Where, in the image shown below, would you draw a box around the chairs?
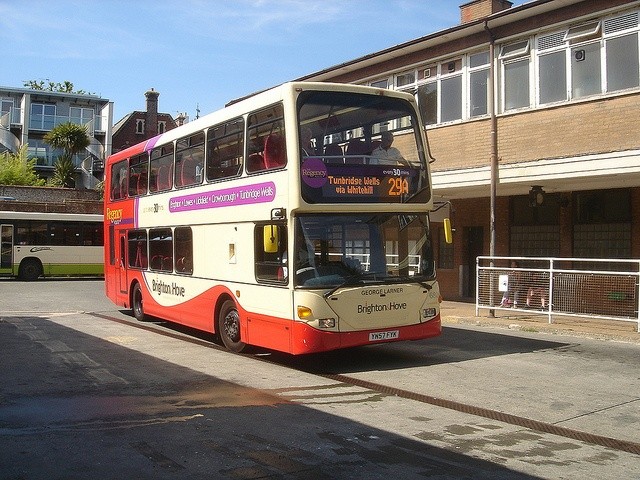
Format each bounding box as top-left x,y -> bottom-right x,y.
278,250 -> 287,280
168,162 -> 174,188
136,171 -> 146,195
247,153 -> 266,171
129,173 -> 139,197
263,133 -> 284,168
128,254 -> 193,272
156,165 -> 170,191
120,177 -> 129,197
111,183 -> 120,199
180,157 -> 194,186
300,127 -> 382,165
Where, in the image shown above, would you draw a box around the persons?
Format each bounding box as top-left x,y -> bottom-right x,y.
370,131 -> 410,165
283,234 -> 311,280
500,260 -> 518,307
299,127 -> 315,154
525,273 -> 546,309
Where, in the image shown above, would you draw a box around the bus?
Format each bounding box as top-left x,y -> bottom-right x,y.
0,210 -> 104,282
103,80 -> 456,358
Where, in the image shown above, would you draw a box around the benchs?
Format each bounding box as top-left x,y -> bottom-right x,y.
498,287 -> 559,311
